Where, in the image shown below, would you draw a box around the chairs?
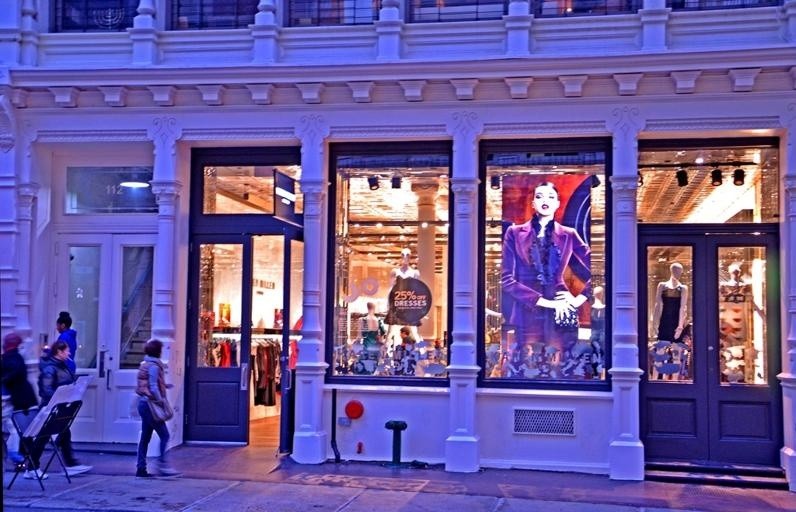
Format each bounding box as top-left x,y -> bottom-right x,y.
7,397 -> 84,492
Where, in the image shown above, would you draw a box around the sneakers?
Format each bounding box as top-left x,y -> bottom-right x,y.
24,468 -> 48,480
63,464 -> 93,476
136,469 -> 183,480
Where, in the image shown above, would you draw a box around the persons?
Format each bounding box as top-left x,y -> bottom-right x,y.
23,310 -> 95,479
0,330 -> 37,463
395,325 -> 418,376
502,181 -> 591,360
373,248 -> 431,379
592,286 -> 606,345
136,338 -> 185,479
719,264 -> 766,342
351,302 -> 388,376
650,263 -> 688,382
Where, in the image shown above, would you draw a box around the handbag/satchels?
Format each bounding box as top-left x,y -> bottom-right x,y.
148,398 -> 173,421
